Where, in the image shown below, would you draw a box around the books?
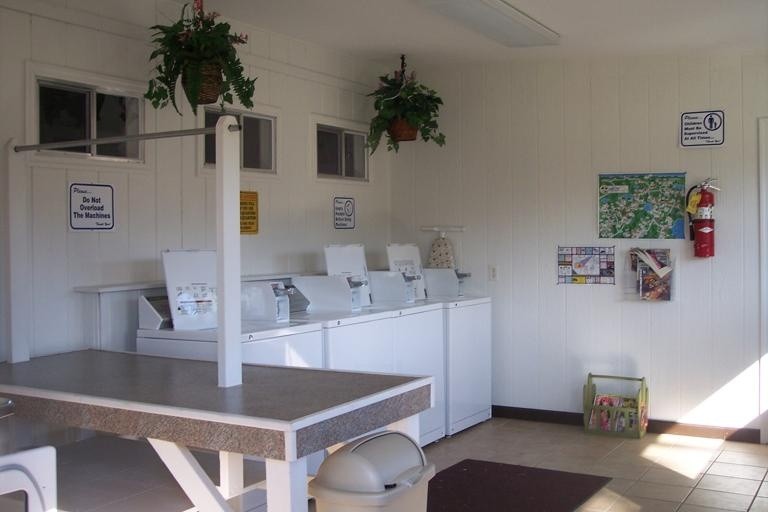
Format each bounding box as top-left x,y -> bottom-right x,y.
615,396 -> 638,431
630,246 -> 673,302
587,394 -> 620,431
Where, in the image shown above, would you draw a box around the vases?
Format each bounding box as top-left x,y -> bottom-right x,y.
386,115 -> 418,142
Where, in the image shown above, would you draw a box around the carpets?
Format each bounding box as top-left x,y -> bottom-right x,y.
427,458 -> 613,512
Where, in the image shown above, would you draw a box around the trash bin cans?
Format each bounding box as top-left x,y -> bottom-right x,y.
307,430 -> 437,512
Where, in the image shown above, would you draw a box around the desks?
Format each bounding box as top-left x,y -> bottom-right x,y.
0,348 -> 436,512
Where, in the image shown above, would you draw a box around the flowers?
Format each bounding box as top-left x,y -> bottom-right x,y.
143,3 -> 258,118
364,64 -> 446,156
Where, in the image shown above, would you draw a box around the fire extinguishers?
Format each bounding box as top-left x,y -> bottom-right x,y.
685,176 -> 721,257
